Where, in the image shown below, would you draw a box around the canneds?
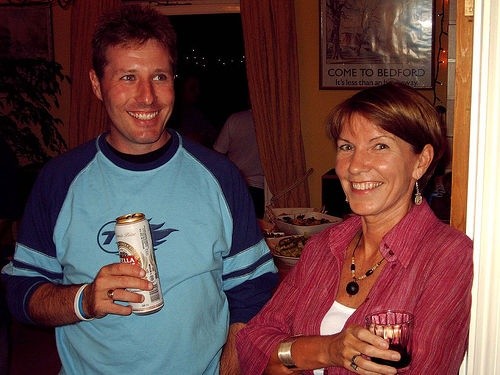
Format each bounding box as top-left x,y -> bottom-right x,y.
115,213 -> 164,314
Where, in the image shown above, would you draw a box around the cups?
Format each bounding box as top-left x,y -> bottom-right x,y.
364,311 -> 415,373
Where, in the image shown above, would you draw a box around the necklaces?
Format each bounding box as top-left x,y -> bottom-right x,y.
347,232 -> 385,297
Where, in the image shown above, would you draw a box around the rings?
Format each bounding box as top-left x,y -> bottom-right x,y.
350,363 -> 359,371
352,354 -> 361,362
107,290 -> 115,300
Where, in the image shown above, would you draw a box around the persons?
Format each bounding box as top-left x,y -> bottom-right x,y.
212,100 -> 265,220
234,82 -> 473,375
1,6 -> 279,375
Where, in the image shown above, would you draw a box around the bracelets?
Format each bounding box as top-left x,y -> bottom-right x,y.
276,335 -> 300,368
73,283 -> 95,321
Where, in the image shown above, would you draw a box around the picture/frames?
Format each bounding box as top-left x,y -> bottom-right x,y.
318,0 -> 435,90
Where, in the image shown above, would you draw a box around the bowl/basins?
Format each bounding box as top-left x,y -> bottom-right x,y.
276,212 -> 344,238
269,235 -> 315,266
268,206 -> 322,217
